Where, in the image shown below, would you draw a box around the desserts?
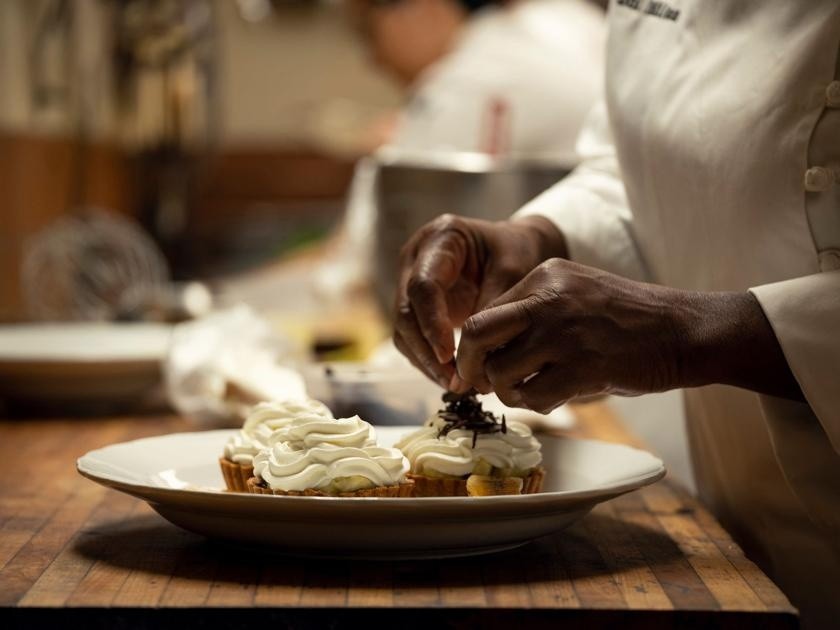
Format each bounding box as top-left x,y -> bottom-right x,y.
219,387 -> 543,498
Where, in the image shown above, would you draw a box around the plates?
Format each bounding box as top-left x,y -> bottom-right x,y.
76,424 -> 668,563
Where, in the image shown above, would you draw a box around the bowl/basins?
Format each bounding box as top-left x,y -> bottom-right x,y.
303,360 -> 437,424
0,320 -> 173,407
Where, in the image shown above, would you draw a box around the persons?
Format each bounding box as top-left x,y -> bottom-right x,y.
389,0 -> 840,630
220,0 -> 692,496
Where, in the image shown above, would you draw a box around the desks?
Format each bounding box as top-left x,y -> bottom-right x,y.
0,334 -> 802,630
